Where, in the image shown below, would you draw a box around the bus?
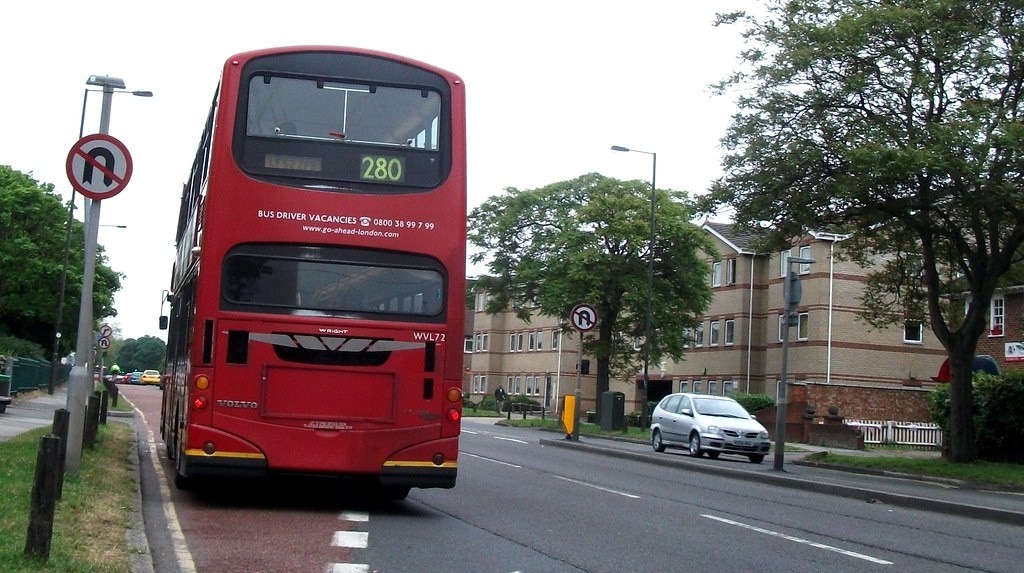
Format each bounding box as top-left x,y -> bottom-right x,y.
159,45 -> 465,507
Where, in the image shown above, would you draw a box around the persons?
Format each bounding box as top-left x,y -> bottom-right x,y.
109,363 -> 120,380
493,384 -> 505,414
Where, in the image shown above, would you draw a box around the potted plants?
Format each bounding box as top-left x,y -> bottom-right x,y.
990,325 -> 1001,335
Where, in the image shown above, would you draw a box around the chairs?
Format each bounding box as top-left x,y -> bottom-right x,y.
279,122 -> 297,135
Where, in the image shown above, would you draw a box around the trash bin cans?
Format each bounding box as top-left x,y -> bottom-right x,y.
602,391 -> 625,433
0,375 -> 11,414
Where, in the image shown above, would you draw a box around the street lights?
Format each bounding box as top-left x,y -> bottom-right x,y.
775,256 -> 817,468
610,145 -> 656,431
64,75 -> 125,474
48,87 -> 152,393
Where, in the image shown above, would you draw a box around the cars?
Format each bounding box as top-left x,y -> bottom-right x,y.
105,374 -> 129,383
129,372 -> 143,383
650,392 -> 772,463
140,369 -> 160,384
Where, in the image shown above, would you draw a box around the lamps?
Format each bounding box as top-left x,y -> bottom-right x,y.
661,362 -> 666,378
